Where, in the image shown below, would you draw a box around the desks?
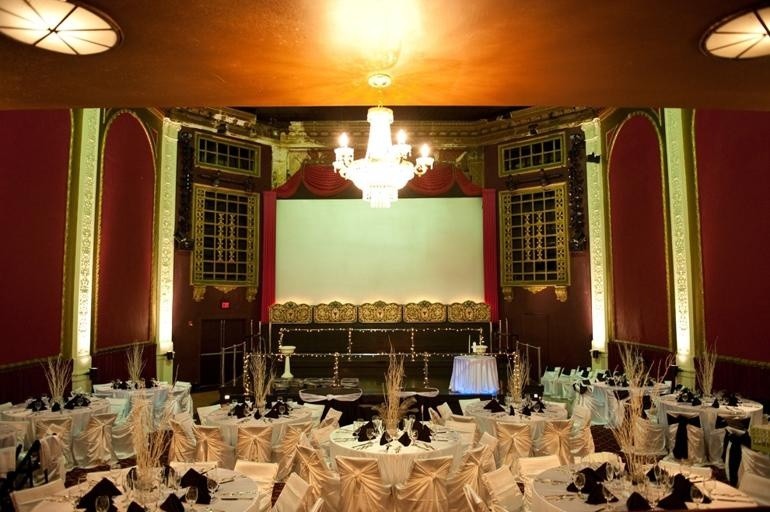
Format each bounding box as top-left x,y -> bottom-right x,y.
447,356 -> 502,395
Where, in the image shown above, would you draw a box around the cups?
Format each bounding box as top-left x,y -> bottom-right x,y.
606,460 -> 614,482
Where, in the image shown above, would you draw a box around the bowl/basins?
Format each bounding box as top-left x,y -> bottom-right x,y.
279,346 -> 296,353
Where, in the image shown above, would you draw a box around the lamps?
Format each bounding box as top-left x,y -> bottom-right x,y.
0,0 -> 125,58
697,1 -> 770,62
332,73 -> 436,212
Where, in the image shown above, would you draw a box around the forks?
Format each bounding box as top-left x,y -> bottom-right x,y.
535,464 -> 576,504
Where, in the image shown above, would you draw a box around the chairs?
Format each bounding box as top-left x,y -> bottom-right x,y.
0,365 -> 770,511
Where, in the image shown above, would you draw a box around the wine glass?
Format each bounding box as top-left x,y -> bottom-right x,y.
602,482 -> 614,512
573,472 -> 586,499
22,377 -> 159,416
467,391 -> 561,417
333,415 -> 453,451
228,396 -> 294,422
45,457 -> 256,511
618,457 -> 750,512
